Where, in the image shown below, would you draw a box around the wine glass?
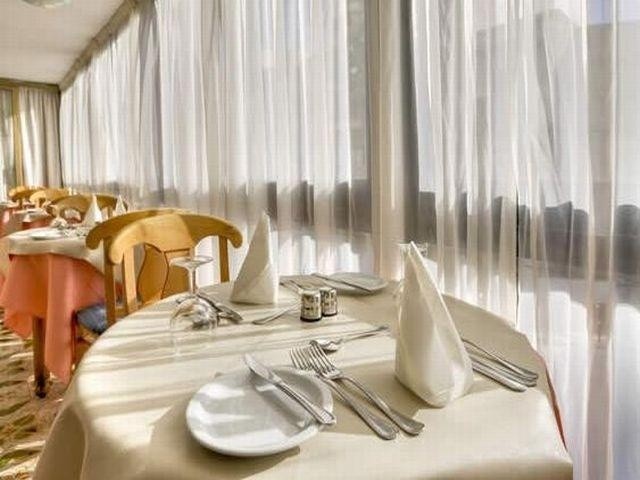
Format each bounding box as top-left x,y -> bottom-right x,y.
48,204 -> 69,230
167,254 -> 217,353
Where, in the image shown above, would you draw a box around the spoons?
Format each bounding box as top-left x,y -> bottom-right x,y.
307,325 -> 390,349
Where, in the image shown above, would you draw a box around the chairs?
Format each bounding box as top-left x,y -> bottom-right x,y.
110,214 -> 244,320
0,182 -> 127,397
69,208 -> 195,366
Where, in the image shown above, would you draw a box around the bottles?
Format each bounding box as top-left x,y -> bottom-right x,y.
298,287 -> 339,323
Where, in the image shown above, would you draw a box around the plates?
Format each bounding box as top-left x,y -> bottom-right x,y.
184,365 -> 335,458
31,230 -> 60,239
324,272 -> 386,296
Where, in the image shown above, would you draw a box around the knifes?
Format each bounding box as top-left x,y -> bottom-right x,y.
244,353 -> 337,428
196,292 -> 245,322
315,272 -> 373,293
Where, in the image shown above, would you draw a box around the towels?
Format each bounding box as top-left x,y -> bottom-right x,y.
394,241 -> 474,408
231,210 -> 283,307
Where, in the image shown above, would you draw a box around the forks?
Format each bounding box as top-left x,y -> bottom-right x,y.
302,342 -> 426,436
287,349 -> 399,440
